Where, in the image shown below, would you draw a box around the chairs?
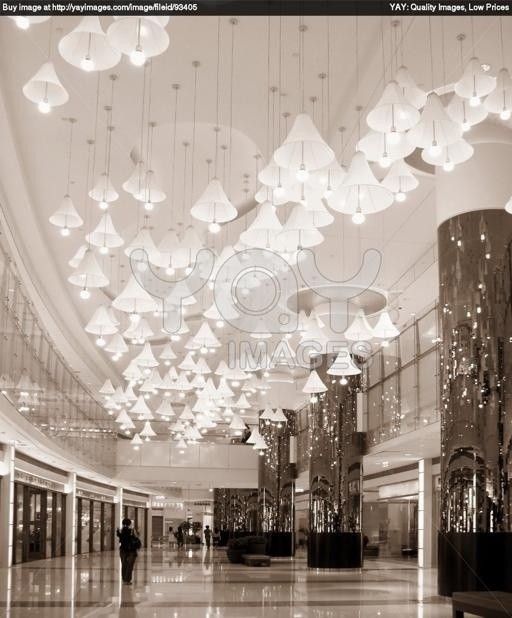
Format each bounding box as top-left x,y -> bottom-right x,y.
363,545 -> 378,557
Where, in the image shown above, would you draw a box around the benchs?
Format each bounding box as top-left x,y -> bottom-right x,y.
452,590 -> 512,618
243,554 -> 272,567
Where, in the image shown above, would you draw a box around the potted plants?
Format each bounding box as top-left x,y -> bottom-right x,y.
226,535 -> 267,562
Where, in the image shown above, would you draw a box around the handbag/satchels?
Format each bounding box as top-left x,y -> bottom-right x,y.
129,537 -> 140,550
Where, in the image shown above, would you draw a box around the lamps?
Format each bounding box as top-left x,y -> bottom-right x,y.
21,12 -> 512,458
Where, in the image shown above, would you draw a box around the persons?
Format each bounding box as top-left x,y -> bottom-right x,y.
203,525 -> 212,549
167,525 -> 176,551
119,518 -> 142,585
212,526 -> 222,549
176,525 -> 184,548
